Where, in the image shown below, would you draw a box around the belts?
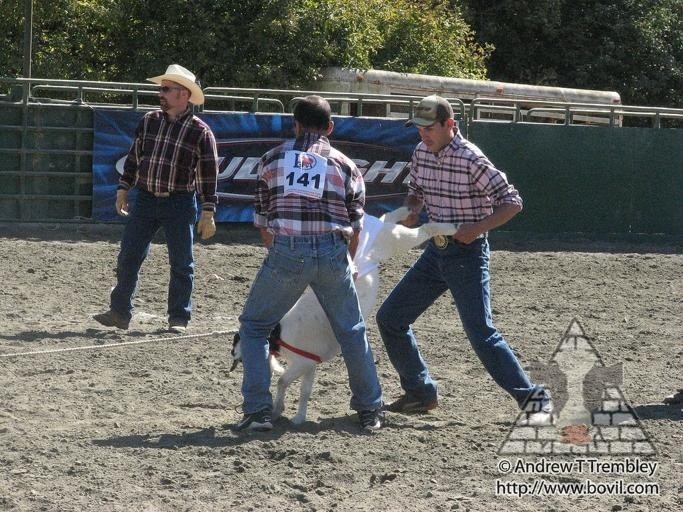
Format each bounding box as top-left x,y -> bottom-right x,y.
139,188 -> 184,199
431,236 -> 456,250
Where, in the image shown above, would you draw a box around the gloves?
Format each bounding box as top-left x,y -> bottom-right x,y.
197,211 -> 216,240
116,191 -> 128,216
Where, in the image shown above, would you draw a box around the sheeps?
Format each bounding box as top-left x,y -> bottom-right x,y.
230,205 -> 486,426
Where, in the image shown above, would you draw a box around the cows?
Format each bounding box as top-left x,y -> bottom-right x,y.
529,357 -> 624,446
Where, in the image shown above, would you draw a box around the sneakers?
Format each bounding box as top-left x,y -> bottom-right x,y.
93,311 -> 129,330
233,409 -> 273,432
360,402 -> 385,431
388,397 -> 438,412
170,323 -> 186,334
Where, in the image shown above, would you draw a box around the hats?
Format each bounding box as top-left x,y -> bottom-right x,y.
146,64 -> 205,106
404,94 -> 453,127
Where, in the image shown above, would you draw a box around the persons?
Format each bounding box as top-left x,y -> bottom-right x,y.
233,94 -> 384,432
375,93 -> 554,426
93,63 -> 217,333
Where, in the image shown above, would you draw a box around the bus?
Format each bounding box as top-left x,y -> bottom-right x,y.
296,63 -> 627,129
